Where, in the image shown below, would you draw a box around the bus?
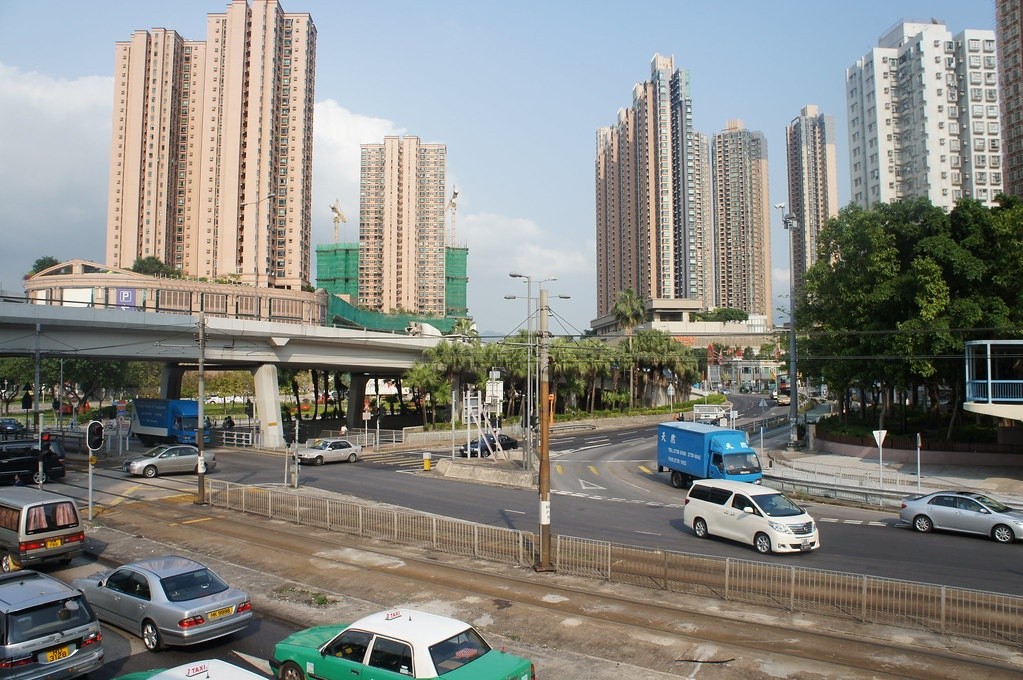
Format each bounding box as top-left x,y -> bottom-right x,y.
776,372 -> 790,405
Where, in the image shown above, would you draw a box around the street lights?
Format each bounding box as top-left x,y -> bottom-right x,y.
504,272 -> 570,468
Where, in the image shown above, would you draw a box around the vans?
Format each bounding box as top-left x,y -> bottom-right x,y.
0,485 -> 84,573
0,439 -> 66,484
683,479 -> 821,554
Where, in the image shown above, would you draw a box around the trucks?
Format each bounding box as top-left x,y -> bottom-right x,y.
655,422 -> 764,490
132,397 -> 210,448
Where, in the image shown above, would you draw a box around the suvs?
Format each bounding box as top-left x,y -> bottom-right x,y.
0,568 -> 103,678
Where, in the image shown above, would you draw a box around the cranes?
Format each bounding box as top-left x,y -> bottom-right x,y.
444,184 -> 460,245
327,199 -> 346,243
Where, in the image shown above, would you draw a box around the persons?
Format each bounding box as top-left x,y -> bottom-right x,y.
222,416 -> 235,431
71,420 -> 74,430
720,457 -> 735,471
169,449 -> 176,457
960,499 -> 979,512
204,416 -> 210,428
341,423 -> 348,436
109,417 -> 117,430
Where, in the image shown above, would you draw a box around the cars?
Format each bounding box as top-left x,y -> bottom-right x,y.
72,554 -> 536,680
898,490 -> 1023,544
121,444 -> 216,478
293,438 -> 361,466
0,418 -> 23,434
459,434 -> 519,458
697,407 -> 726,426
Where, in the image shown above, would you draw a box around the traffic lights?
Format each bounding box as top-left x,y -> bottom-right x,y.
41,431 -> 51,458
86,421 -> 104,451
530,416 -> 539,429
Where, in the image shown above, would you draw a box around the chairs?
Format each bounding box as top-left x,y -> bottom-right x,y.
166,582 -> 177,592
16,617 -> 34,634
57,608 -> 73,621
197,575 -> 209,586
